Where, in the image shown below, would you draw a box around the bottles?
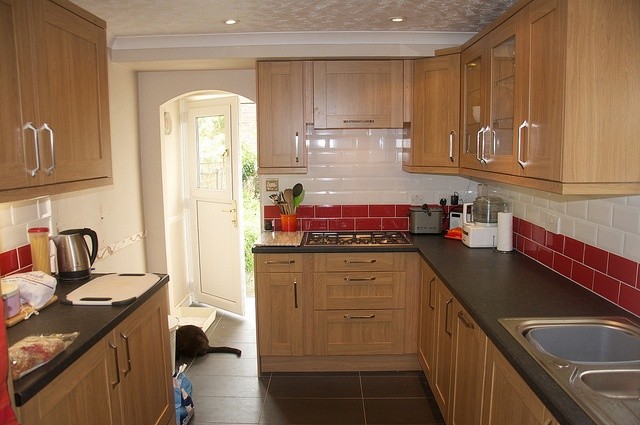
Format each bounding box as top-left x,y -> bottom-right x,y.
265,220 -> 272,230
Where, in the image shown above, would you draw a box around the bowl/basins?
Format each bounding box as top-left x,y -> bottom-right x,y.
472,106 -> 481,123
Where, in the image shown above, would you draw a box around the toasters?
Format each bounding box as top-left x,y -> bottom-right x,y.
409,204 -> 443,236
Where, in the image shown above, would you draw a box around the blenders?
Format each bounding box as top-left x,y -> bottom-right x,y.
462,184 -> 504,249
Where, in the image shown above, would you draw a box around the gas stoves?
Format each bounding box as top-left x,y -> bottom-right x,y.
301,232 -> 413,248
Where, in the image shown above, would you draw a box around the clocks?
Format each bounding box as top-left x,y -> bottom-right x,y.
164,112 -> 173,134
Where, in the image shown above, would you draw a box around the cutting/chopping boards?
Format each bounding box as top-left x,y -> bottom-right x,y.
5,294 -> 58,327
58,273 -> 162,305
254,230 -> 304,247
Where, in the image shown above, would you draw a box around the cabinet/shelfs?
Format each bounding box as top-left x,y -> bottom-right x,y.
517,1 -> 640,195
303,253 -> 418,372
438,276 -> 454,425
255,254 -> 303,378
418,252 -> 437,405
1,1 -> 113,204
487,336 -> 560,424
454,298 -> 487,425
313,61 -> 403,129
17,284 -> 176,424
403,46 -> 461,174
461,1 -> 517,185
256,58 -> 313,175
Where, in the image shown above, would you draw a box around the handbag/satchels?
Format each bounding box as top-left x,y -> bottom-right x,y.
172,364 -> 194,425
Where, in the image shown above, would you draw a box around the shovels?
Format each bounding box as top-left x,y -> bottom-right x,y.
284,189 -> 294,214
294,190 -> 306,214
293,183 -> 303,197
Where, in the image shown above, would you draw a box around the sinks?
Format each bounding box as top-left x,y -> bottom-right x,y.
577,367 -> 640,400
517,320 -> 640,363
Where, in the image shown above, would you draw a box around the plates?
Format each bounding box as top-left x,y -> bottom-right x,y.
444,235 -> 462,240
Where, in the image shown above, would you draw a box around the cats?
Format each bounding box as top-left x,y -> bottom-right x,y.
175,324 -> 242,361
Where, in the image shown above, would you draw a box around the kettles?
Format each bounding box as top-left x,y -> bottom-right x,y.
49,228 -> 98,281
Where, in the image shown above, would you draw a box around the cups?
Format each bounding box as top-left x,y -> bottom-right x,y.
28,228 -> 51,275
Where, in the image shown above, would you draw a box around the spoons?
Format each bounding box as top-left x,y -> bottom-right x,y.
276,201 -> 289,214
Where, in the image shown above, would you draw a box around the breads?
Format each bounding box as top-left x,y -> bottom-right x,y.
20,335 -> 64,362
9,345 -> 34,378
